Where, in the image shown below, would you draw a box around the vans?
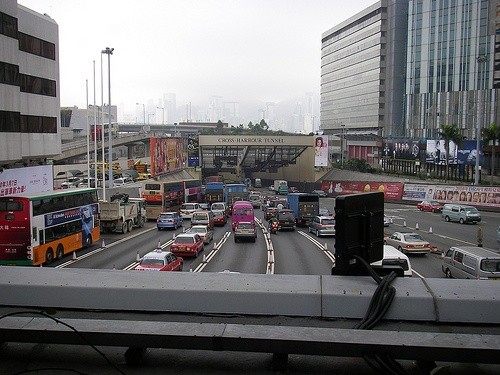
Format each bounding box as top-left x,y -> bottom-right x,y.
371,244 -> 412,279
113,177 -> 135,187
179,202 -> 203,219
190,211 -> 216,230
441,245 -> 500,281
442,203 -> 481,224
211,202 -> 229,218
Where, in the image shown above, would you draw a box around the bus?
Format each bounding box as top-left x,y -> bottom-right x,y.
286,192 -> 320,227
230,200 -> 255,230
134,162 -> 148,180
141,178 -> 201,219
0,187 -> 102,267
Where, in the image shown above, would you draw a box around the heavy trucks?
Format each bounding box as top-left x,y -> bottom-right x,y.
89,162 -> 121,181
206,181 -> 224,202
226,184 -> 250,213
99,196 -> 147,235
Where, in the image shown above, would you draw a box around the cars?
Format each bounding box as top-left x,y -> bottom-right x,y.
385,231 -> 431,256
61,176 -> 98,189
234,221 -> 256,243
245,177 -> 393,237
214,212 -> 228,226
135,248 -> 184,272
200,204 -> 212,211
417,199 -> 445,214
170,225 -> 213,258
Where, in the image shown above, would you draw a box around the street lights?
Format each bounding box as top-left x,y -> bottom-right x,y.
148,113 -> 155,124
101,46 -> 115,189
475,52 -> 490,186
136,102 -> 146,124
157,106 -> 165,124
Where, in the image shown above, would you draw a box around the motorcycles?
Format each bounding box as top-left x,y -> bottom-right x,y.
268,221 -> 278,234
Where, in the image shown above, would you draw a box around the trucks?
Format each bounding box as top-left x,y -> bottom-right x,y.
273,179 -> 289,195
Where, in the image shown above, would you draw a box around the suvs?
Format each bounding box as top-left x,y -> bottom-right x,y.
156,211 -> 184,230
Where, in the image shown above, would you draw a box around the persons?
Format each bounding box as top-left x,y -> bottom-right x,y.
428,189 -> 500,203
268,215 -> 278,230
432,141 -> 440,163
394,142 -> 409,156
315,137 -> 323,156
